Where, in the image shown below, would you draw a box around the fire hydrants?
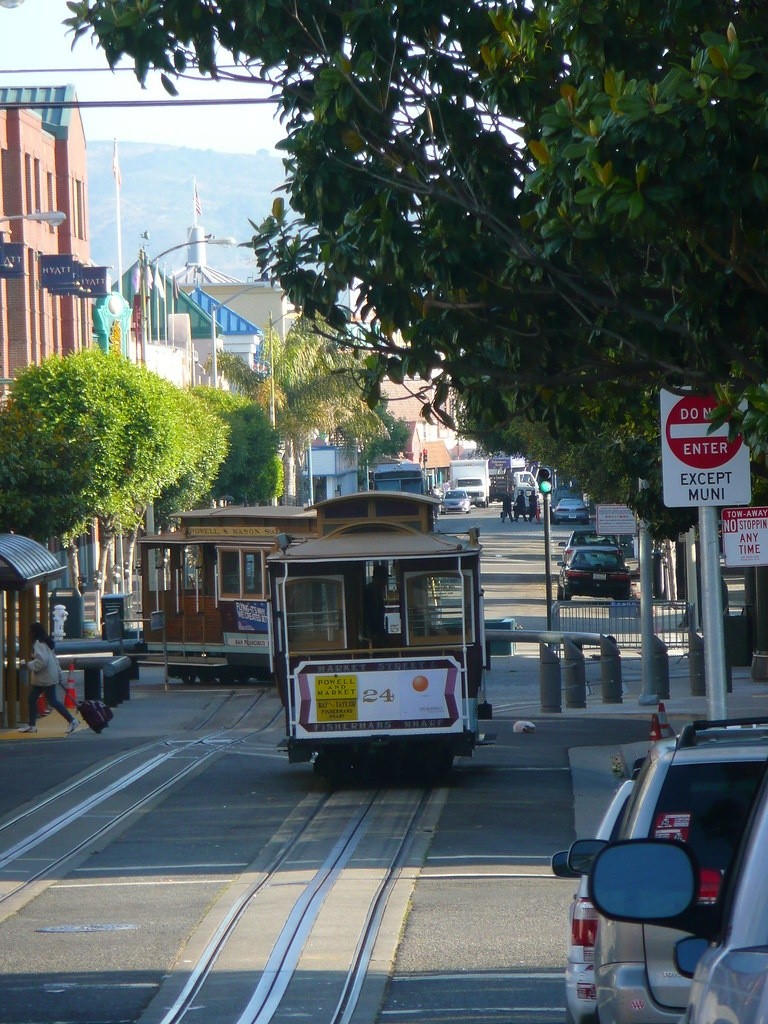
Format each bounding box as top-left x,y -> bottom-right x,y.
51,604 -> 69,641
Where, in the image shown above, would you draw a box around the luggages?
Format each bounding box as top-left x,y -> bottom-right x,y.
59,683 -> 113,734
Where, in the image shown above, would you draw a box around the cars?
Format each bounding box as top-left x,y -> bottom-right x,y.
513,487 -> 590,524
551,768 -> 641,1024
557,546 -> 631,601
557,529 -> 625,563
587,773 -> 766,1024
441,490 -> 472,515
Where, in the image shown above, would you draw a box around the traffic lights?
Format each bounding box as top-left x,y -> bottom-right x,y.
423,450 -> 427,463
538,467 -> 553,494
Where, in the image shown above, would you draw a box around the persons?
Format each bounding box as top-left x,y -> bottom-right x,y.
18,621 -> 80,734
502,490 -> 539,523
364,566 -> 391,658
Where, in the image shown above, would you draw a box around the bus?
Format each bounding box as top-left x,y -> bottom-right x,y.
136,491 -> 492,786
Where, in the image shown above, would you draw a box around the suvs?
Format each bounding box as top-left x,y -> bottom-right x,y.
567,714 -> 768,1024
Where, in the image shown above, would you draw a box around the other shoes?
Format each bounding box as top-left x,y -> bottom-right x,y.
64,718 -> 81,733
17,726 -> 37,733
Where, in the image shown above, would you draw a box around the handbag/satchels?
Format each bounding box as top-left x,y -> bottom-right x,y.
513,502 -> 517,511
500,512 -> 507,518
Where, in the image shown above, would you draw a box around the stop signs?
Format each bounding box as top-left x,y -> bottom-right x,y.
665,396 -> 743,470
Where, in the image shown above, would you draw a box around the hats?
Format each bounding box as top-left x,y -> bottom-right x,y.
371,565 -> 392,578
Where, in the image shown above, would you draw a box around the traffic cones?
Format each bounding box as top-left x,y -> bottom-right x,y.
63,663 -> 78,708
658,703 -> 675,738
38,692 -> 47,715
649,713 -> 664,751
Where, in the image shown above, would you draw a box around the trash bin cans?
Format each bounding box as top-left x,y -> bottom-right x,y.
48,588 -> 85,638
101,592 -> 131,639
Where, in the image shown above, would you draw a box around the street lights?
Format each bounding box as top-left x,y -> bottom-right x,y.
211,283 -> 281,387
142,238 -> 236,364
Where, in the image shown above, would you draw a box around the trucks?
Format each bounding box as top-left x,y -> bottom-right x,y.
369,464 -> 426,495
449,459 -> 539,508
